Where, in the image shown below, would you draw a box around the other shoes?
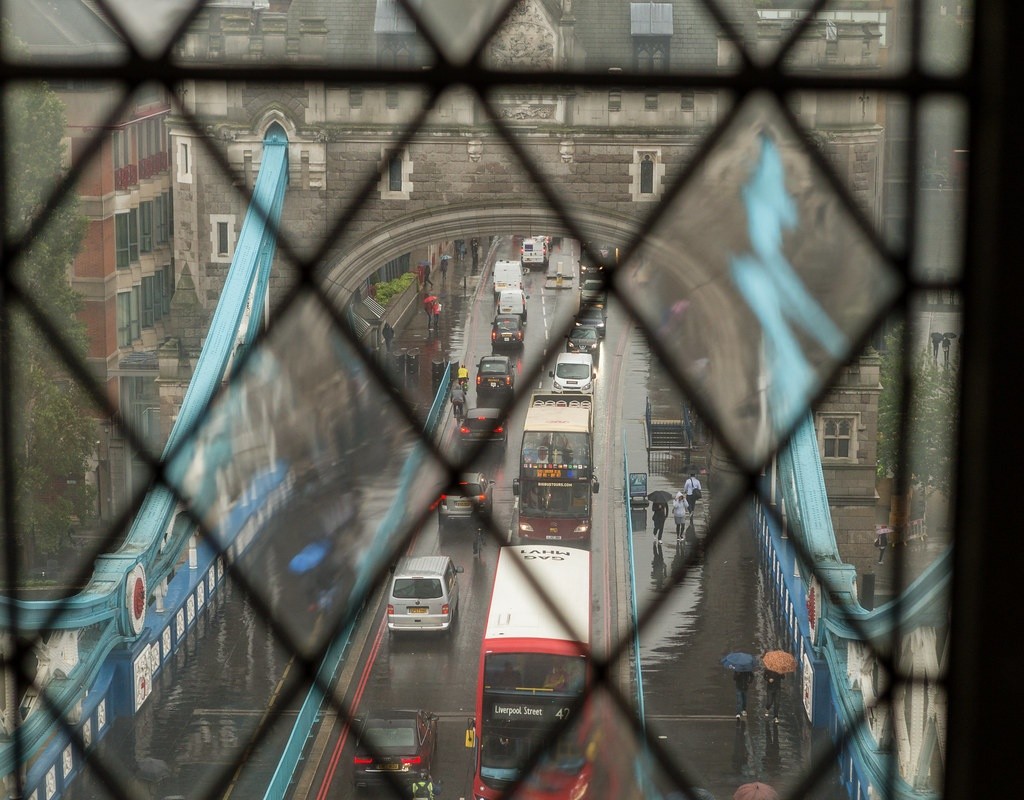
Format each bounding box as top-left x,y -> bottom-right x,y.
460,417 -> 463,422
453,413 -> 456,418
654,535 -> 656,540
431,284 -> 433,287
690,512 -> 693,518
736,714 -> 741,717
680,535 -> 683,539
659,539 -> 663,544
765,709 -> 768,716
424,283 -> 426,286
774,717 -> 779,723
742,711 -> 747,716
677,536 -> 679,540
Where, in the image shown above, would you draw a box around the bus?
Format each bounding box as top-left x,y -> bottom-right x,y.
513,392 -> 599,545
465,545 -> 601,800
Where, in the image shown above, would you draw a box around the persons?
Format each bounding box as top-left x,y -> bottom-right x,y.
457,364 -> 469,391
684,473 -> 701,515
441,259 -> 448,279
416,264 -> 434,288
878,533 -> 887,565
382,322 -> 394,353
525,485 -> 539,509
534,446 -> 553,463
763,669 -> 786,724
493,661 -> 522,689
541,665 -> 566,691
489,721 -> 515,767
732,671 -> 755,719
405,768 -> 442,800
455,239 -> 466,262
470,237 -> 478,256
450,383 -> 466,418
672,490 -> 690,541
425,300 -> 442,328
651,501 -> 669,544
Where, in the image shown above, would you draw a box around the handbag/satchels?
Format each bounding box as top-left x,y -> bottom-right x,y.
693,489 -> 702,500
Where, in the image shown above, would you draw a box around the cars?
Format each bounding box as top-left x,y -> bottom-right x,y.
491,315 -> 527,348
351,707 -> 440,789
563,251 -> 609,356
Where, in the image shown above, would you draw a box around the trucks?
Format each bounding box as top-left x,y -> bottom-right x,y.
491,260 -> 527,295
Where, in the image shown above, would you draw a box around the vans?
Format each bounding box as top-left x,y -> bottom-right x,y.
519,237 -> 549,268
496,289 -> 531,318
547,351 -> 597,397
386,556 -> 464,638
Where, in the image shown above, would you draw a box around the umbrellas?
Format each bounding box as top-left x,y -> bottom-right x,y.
678,463 -> 711,476
440,254 -> 452,259
876,528 -> 895,534
733,781 -> 779,800
666,786 -> 715,800
415,258 -> 432,266
648,490 -> 673,503
762,650 -> 797,673
423,295 -> 438,304
721,652 -> 761,672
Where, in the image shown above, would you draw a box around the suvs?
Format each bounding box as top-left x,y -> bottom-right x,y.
457,407 -> 510,453
438,472 -> 496,527
475,355 -> 516,398
